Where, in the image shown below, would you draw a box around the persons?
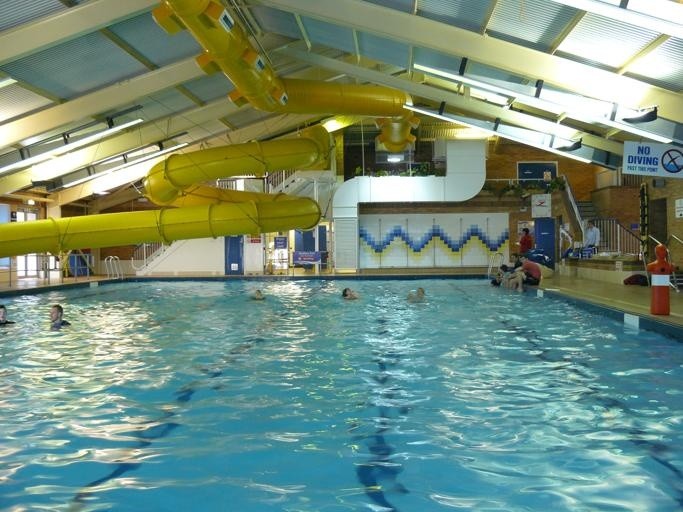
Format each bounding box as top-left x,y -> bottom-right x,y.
582,220 -> 601,254
647,244 -> 675,316
0,304 -> 16,328
255,289 -> 264,300
490,253 -> 541,293
407,286 -> 426,302
519,229 -> 532,253
342,287 -> 358,300
49,305 -> 71,330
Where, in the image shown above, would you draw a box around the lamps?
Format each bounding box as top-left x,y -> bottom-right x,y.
554,139 -> 581,151
622,107 -> 657,124
